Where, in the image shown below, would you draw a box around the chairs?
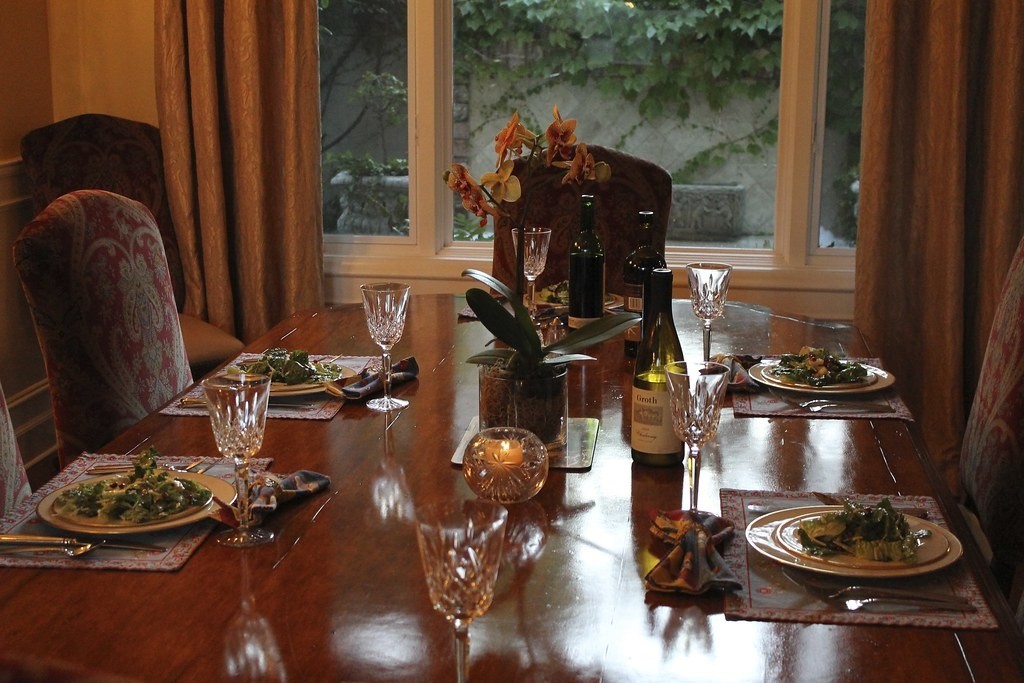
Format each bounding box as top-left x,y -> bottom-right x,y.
12,189 -> 195,465
489,146 -> 670,302
956,233 -> 1024,615
23,114 -> 243,378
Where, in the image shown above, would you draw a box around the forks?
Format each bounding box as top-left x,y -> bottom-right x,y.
781,565 -> 977,611
88,456 -> 221,474
767,388 -> 896,413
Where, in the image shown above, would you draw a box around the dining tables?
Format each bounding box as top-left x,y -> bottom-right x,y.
0,289 -> 1023,683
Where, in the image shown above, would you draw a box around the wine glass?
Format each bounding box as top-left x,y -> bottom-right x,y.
201,373 -> 274,544
686,262 -> 732,364
359,282 -> 411,411
664,361 -> 730,514
413,499 -> 507,683
511,227 -> 552,326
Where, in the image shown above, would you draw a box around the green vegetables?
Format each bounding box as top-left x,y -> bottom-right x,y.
793,494 -> 932,561
50,443 -> 212,523
226,346 -> 341,385
768,347 -> 868,387
537,277 -> 615,306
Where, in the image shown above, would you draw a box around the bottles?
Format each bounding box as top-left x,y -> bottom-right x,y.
631,269 -> 687,465
530,195 -> 667,357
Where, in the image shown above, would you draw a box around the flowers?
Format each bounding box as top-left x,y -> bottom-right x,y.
443,106 -> 642,377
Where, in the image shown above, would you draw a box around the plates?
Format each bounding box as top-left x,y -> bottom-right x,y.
522,291 -> 626,309
269,360 -> 356,397
745,506 -> 963,576
37,469 -> 238,534
749,360 -> 896,393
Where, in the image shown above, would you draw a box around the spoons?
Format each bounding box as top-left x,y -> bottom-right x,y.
0,539 -> 106,556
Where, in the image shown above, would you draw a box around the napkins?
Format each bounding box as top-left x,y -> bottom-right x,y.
642,509 -> 742,594
322,355 -> 419,402
209,468 -> 330,532
710,351 -> 765,392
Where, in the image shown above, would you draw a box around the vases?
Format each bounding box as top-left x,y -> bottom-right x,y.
479,361 -> 570,462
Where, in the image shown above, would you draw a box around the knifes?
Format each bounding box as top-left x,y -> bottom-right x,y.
0,533 -> 168,553
180,397 -> 313,409
